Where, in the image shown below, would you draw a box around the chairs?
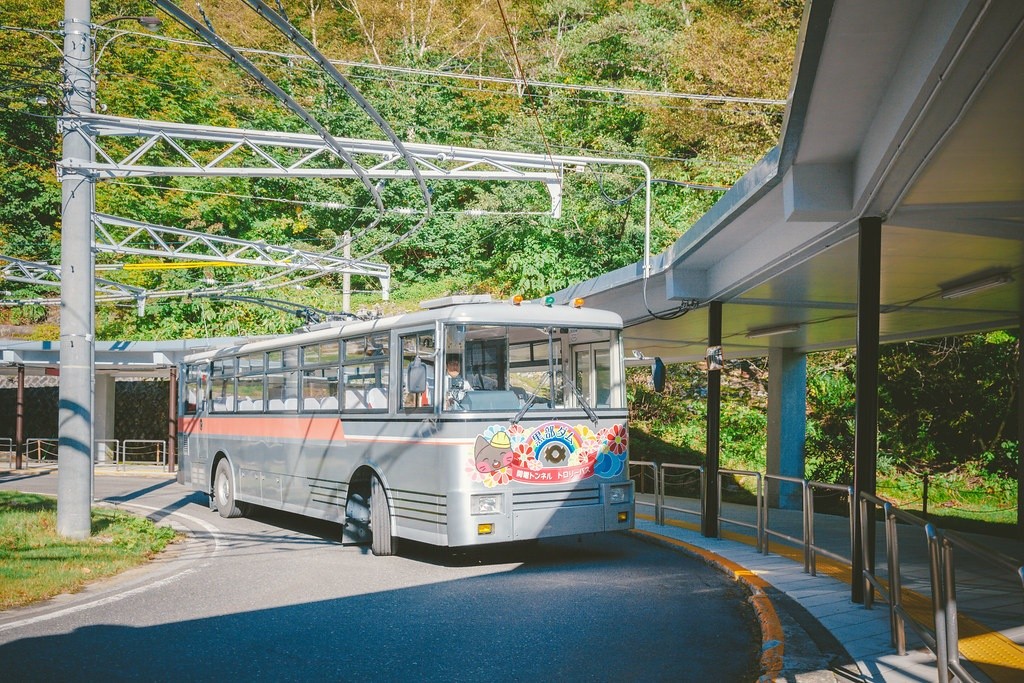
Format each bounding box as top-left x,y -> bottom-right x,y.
212,387 -> 388,413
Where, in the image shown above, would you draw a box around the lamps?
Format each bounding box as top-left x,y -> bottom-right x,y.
942,274 -> 1016,300
746,326 -> 800,339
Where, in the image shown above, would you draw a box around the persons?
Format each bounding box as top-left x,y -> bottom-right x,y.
444,358 -> 474,411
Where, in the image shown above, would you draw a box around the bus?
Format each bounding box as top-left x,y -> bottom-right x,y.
176,294 -> 667,557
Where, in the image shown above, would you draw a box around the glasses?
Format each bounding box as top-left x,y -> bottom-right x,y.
447,362 -> 460,366
